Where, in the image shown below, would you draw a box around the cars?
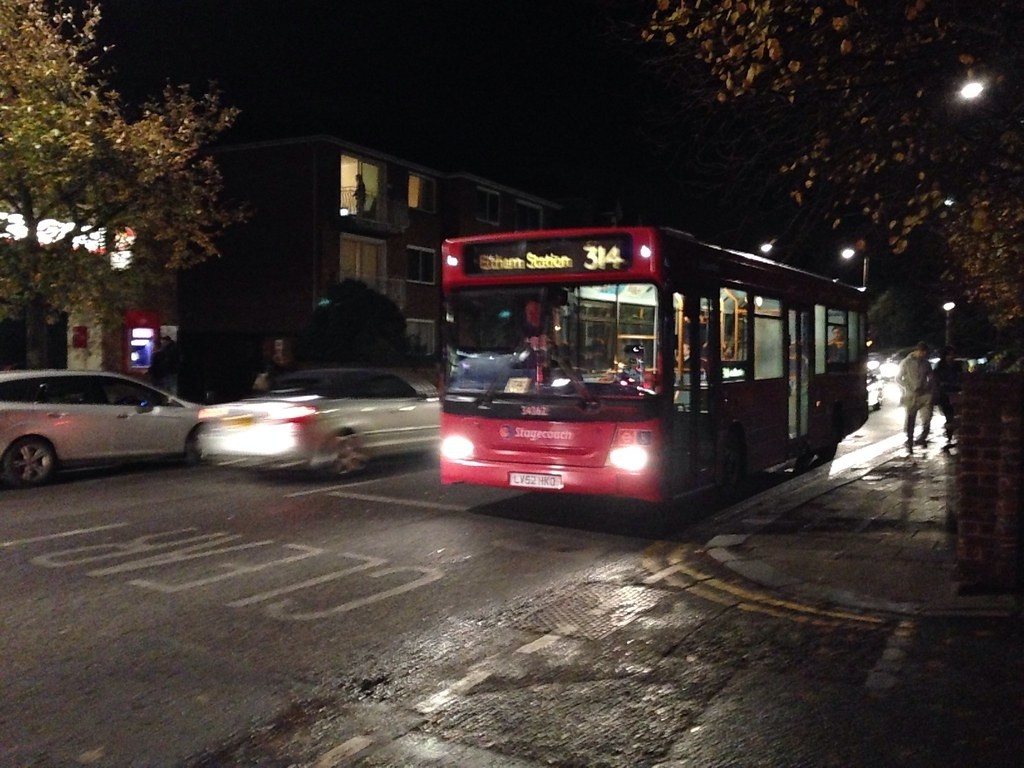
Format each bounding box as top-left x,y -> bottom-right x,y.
199,369 -> 439,477
0,370 -> 207,489
866,344 -> 975,411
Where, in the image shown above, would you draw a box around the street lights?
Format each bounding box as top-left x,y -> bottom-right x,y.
942,301 -> 955,346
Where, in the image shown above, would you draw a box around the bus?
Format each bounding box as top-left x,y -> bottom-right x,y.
439,226 -> 869,504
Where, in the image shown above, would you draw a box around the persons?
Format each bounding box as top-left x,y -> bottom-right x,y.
145,336 -> 189,401
932,345 -> 971,441
353,174 -> 367,213
828,326 -> 846,348
902,342 -> 935,453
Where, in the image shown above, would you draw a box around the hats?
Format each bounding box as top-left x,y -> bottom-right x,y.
916,342 -> 929,350
